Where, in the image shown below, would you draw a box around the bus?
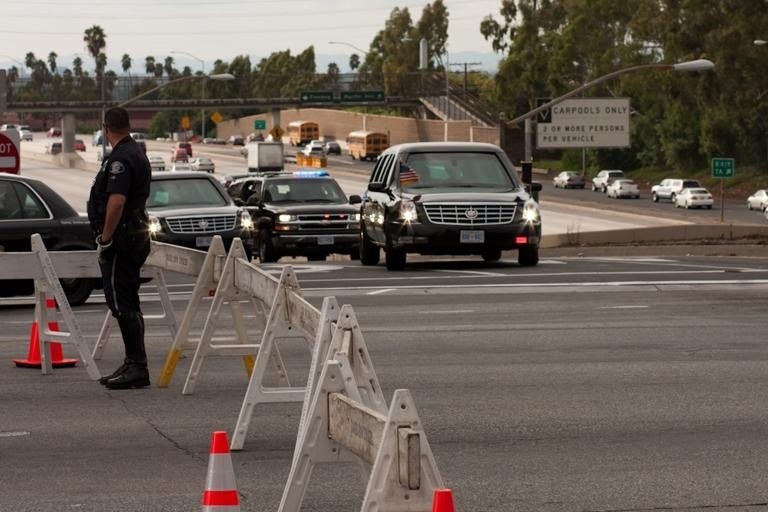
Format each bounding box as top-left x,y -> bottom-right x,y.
287,120 -> 318,146
344,128 -> 388,161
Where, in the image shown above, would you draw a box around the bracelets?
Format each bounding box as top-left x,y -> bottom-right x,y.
95,234 -> 113,247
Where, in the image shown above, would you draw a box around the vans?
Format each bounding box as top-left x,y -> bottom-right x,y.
130,132 -> 144,144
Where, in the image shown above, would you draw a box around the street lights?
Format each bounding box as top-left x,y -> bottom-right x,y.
171,50 -> 204,143
498,58 -> 714,202
399,37 -> 450,119
328,41 -> 368,56
102,73 -> 235,163
754,39 -> 768,46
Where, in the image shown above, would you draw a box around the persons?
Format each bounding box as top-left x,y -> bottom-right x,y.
86,107 -> 151,389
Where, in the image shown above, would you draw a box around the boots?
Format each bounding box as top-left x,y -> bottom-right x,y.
101,312 -> 150,387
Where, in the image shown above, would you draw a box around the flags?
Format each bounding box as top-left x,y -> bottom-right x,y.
399,159 -> 419,186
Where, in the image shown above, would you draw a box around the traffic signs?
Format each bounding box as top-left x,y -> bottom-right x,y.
300,92 -> 333,103
712,157 -> 732,177
254,119 -> 265,129
341,91 -> 384,104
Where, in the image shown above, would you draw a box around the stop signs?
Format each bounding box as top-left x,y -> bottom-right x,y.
0,130 -> 20,174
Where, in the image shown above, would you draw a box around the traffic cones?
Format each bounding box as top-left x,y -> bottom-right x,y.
431,489 -> 454,512
199,431 -> 239,512
12,294 -> 80,365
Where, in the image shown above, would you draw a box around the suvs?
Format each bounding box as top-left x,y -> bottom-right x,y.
219,170 -> 361,261
144,171 -> 254,264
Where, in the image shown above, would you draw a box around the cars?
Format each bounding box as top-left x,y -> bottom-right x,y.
359,141 -> 540,270
227,132 -> 284,171
300,139 -> 341,156
2,124 -> 217,173
552,169 -> 767,219
0,175 -> 153,305
